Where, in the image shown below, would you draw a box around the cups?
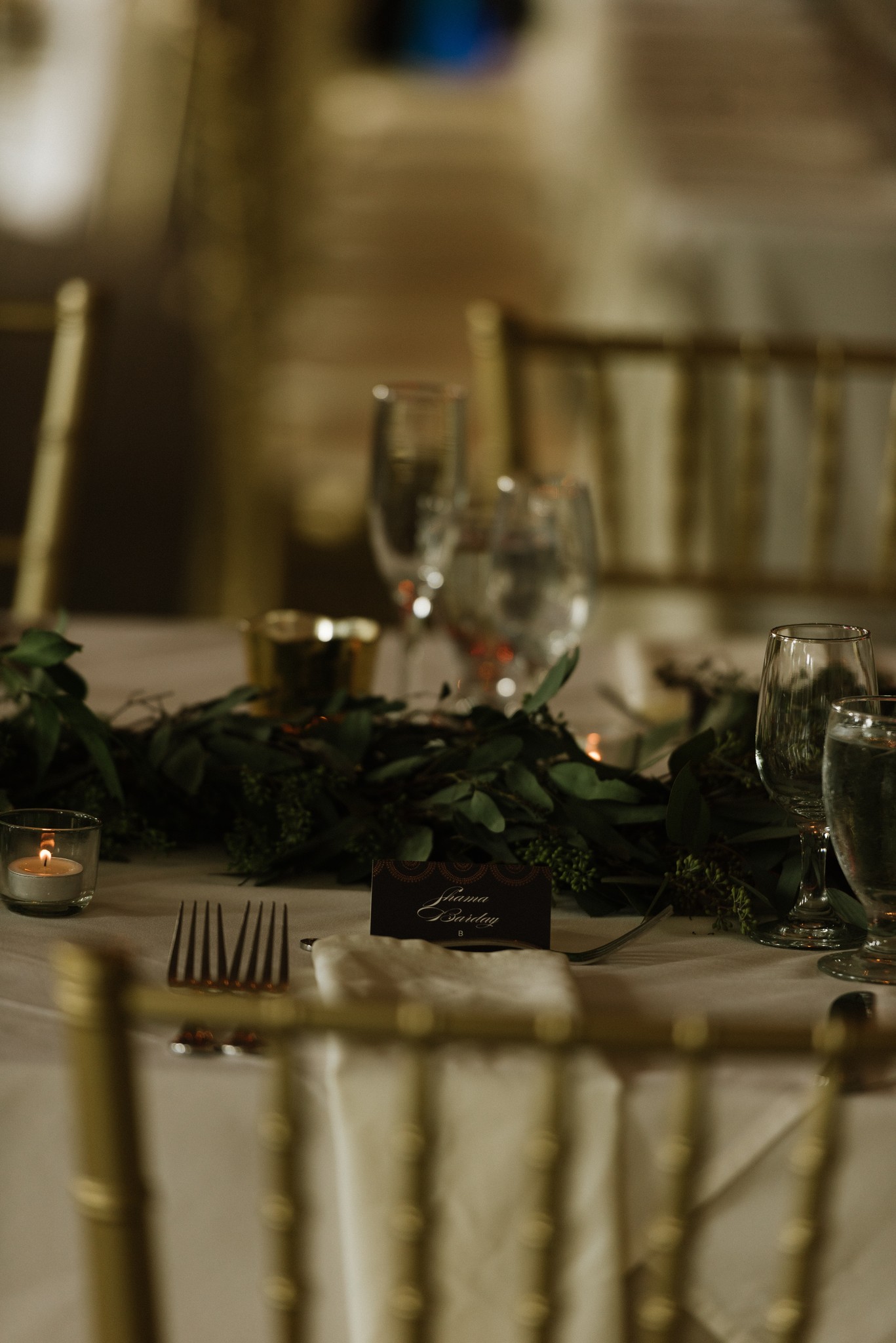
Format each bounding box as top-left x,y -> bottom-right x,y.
0,808 -> 103,918
247,604 -> 382,711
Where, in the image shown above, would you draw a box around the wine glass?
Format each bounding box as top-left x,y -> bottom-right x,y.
817,697 -> 896,984
366,384 -> 467,716
446,478 -> 606,719
749,621 -> 878,951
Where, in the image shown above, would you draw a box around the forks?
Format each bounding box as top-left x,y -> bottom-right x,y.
169,898 -> 230,1058
226,901 -> 293,1058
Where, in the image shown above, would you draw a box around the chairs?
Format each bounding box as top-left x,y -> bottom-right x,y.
52,928 -> 877,1343
461,294 -> 893,666
